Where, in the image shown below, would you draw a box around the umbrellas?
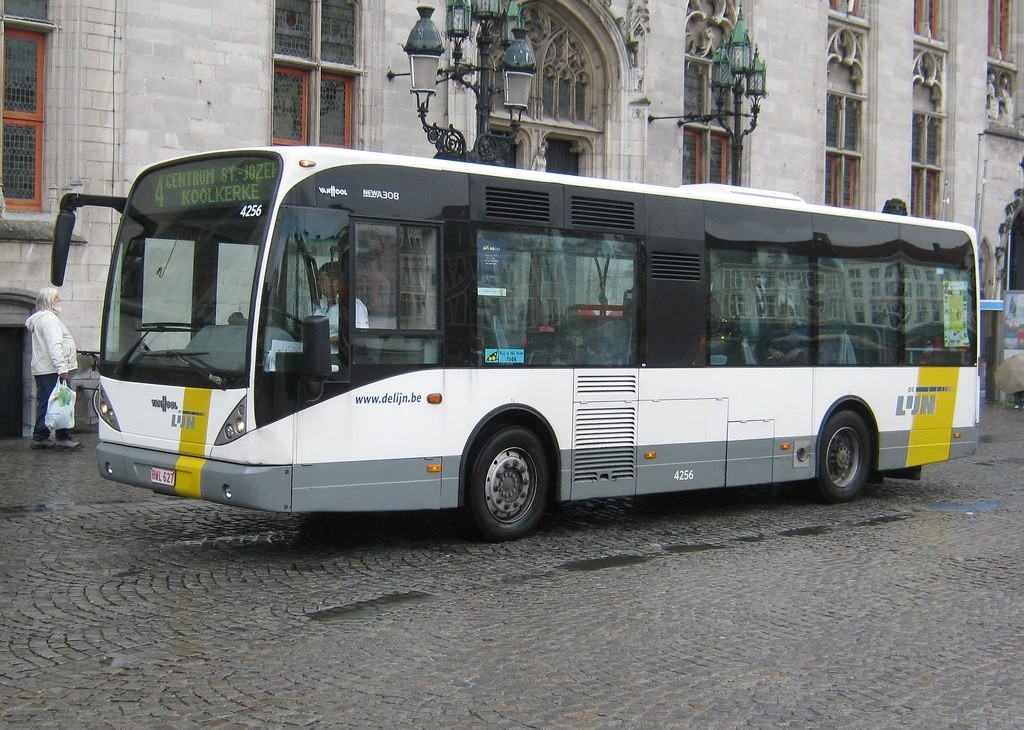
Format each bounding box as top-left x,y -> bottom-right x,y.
994,353 -> 1024,394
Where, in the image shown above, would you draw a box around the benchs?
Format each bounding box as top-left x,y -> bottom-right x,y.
563,315 -> 633,366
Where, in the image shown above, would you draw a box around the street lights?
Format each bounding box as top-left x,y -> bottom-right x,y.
447,0 -> 523,139
709,4 -> 775,194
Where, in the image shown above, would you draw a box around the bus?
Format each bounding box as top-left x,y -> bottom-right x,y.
50,151 -> 982,546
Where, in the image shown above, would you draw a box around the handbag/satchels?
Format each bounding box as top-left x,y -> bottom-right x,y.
45,376 -> 76,430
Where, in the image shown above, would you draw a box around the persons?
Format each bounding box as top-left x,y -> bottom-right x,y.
754,304 -> 809,365
25,288 -> 86,451
314,261 -> 370,373
1013,390 -> 1024,410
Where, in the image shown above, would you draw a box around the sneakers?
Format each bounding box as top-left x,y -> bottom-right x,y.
54,439 -> 85,452
31,438 -> 55,449
